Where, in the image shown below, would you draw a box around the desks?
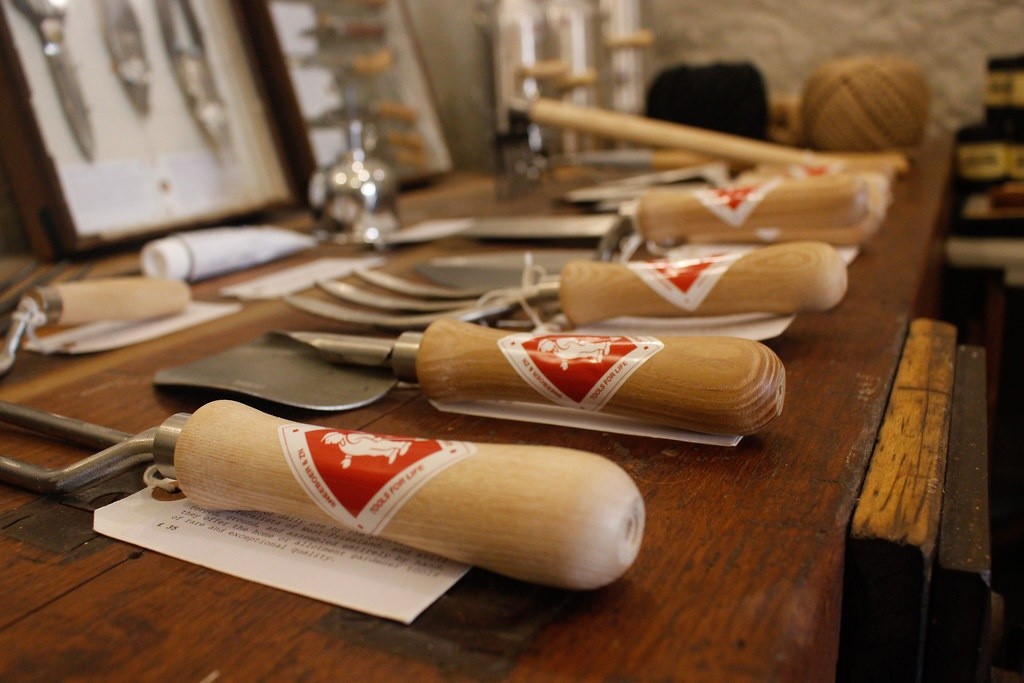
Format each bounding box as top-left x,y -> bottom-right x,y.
0,130 -> 993,683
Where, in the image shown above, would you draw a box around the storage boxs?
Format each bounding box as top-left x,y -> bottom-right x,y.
0,0 -> 458,260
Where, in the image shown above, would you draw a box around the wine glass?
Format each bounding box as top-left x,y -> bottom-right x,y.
309,25 -> 402,239
